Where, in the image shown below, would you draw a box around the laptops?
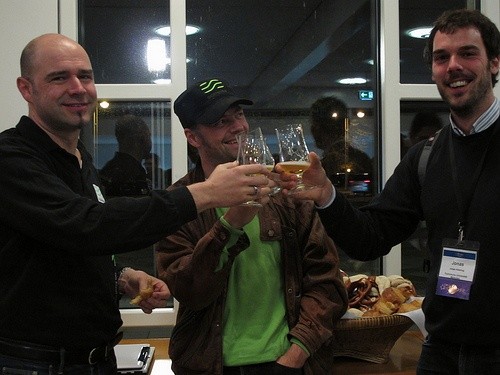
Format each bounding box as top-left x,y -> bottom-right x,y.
113,343 -> 155,375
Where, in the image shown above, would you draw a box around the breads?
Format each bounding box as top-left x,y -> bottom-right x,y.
338,269 -> 421,317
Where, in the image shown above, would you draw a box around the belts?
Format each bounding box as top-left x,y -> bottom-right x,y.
0,330 -> 123,363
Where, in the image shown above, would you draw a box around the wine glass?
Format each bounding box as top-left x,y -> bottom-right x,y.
235,126 -> 281,194
235,133 -> 268,208
275,124 -> 317,195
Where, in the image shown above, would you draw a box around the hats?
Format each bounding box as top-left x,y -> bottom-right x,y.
174,79 -> 252,128
